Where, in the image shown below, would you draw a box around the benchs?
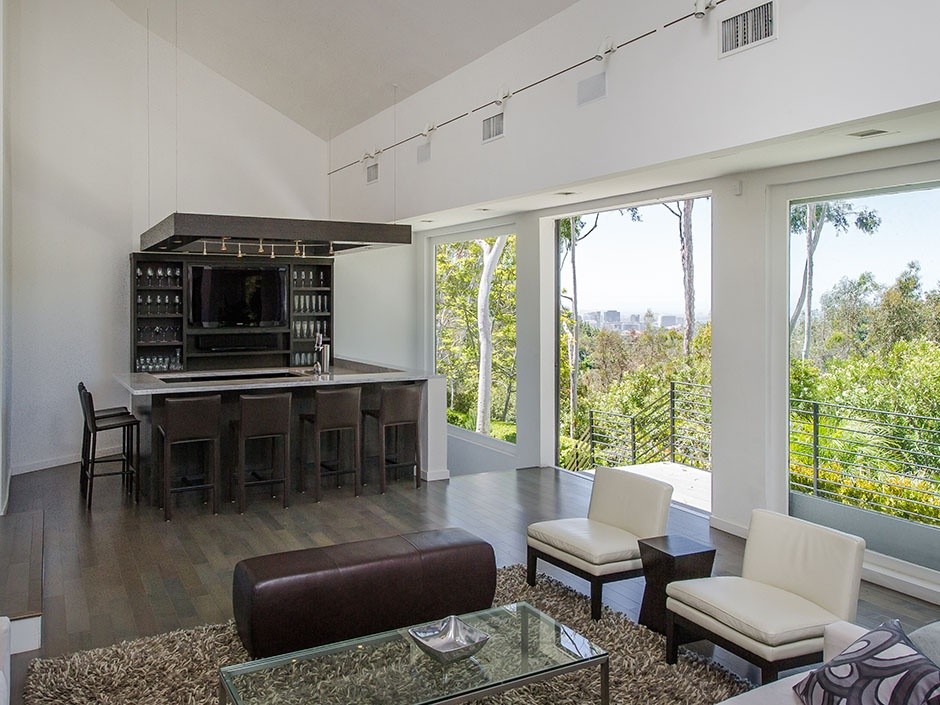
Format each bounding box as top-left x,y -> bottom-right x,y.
527,465 -> 674,622
663,506 -> 866,687
231,526 -> 496,656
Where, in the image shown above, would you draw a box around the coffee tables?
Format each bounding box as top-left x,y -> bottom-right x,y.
218,600 -> 611,705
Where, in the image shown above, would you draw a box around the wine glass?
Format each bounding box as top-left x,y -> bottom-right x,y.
137,294 -> 182,315
136,325 -> 179,343
136,267 -> 180,288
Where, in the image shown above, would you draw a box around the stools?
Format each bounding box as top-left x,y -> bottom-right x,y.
226,383 -> 426,516
78,381 -> 221,528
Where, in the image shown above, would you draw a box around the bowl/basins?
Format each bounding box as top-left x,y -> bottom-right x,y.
409,615 -> 489,665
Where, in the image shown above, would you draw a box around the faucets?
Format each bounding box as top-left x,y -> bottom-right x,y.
314,343 -> 331,375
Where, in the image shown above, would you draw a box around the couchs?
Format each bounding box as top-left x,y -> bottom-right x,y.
712,621 -> 871,705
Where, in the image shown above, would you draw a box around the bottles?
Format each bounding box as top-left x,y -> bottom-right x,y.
293,270 -> 323,288
175,347 -> 184,372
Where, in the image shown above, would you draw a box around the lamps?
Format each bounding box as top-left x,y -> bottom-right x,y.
359,150 -> 370,164
422,119 -> 437,137
694,1 -> 716,19
595,37 -> 617,61
368,144 -> 383,159
495,84 -> 512,105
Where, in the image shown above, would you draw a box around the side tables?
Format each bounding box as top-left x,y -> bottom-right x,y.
636,531 -> 716,645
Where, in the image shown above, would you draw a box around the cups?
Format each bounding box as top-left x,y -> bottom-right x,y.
292,320 -> 327,339
136,354 -> 183,372
293,295 -> 329,313
293,351 -> 319,365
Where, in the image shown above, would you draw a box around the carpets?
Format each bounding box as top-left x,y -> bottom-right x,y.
23,561 -> 758,705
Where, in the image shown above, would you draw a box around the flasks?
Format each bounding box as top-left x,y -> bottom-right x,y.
320,344 -> 331,374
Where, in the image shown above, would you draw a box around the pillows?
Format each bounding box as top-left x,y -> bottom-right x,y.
907,621 -> 940,669
793,617 -> 940,705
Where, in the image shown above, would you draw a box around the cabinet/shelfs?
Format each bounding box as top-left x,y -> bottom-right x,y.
287,258 -> 335,369
130,251 -> 185,375
186,325 -> 291,358
